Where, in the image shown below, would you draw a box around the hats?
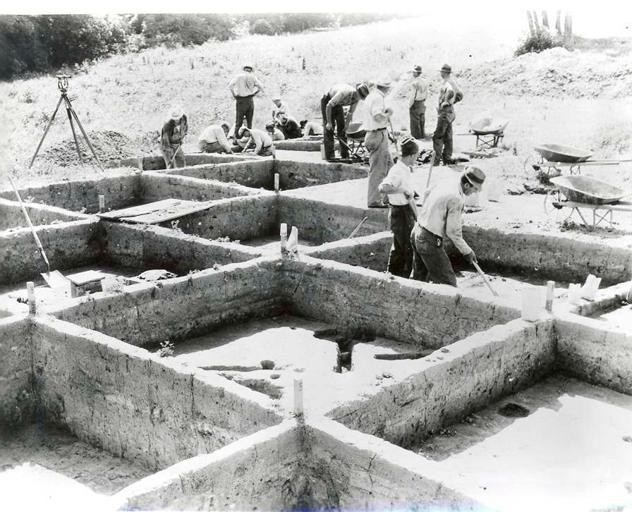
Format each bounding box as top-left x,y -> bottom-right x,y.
238,125 -> 246,136
377,77 -> 391,88
400,139 -> 419,157
272,97 -> 280,102
413,66 -> 421,73
225,122 -> 231,131
170,110 -> 183,120
440,64 -> 450,73
356,83 -> 369,100
464,166 -> 486,192
244,64 -> 252,69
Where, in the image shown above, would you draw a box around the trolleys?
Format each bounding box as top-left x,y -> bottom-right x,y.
346,122 -> 370,155
545,174 -> 632,227
457,117 -> 510,149
533,144 -> 631,176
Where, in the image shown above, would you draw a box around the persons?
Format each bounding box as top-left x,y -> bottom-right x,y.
160,114 -> 188,170
320,83 -> 369,163
431,64 -> 463,166
238,126 -> 276,157
364,79 -> 394,208
265,97 -> 322,142
228,64 -> 262,140
378,136 -> 419,279
410,163 -> 486,285
197,123 -> 234,155
408,64 -> 428,138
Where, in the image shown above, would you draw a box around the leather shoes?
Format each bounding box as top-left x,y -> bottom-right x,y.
341,157 -> 354,163
329,157 -> 340,163
368,204 -> 389,209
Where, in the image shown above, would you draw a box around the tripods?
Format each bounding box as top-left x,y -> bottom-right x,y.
28,95 -> 107,173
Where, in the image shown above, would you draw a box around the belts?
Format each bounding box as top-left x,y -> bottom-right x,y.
415,100 -> 423,103
418,224 -> 438,238
375,127 -> 386,133
389,204 -> 409,208
